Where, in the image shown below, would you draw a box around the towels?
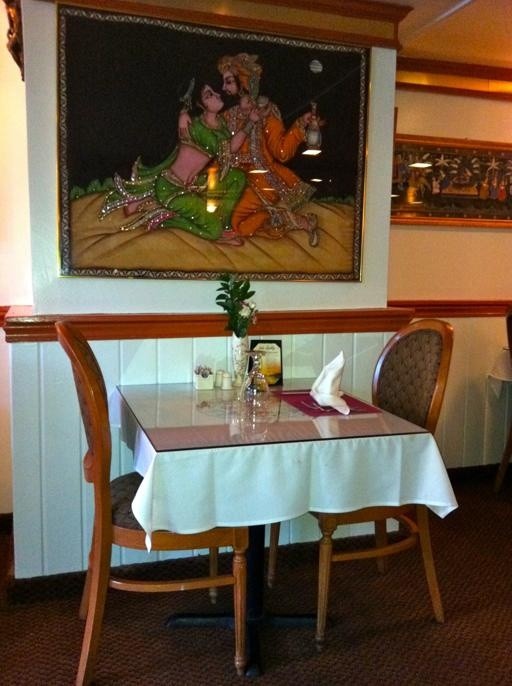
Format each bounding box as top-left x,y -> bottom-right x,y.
310,349 -> 350,415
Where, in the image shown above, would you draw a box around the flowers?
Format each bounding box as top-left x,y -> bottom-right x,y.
211,270 -> 259,337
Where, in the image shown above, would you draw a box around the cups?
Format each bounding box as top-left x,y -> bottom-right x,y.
252,391 -> 279,423
242,415 -> 269,442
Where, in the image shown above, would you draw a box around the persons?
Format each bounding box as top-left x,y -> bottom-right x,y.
179,54 -> 322,248
97,75 -> 261,241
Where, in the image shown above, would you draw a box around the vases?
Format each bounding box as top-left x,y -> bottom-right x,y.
228,332 -> 253,391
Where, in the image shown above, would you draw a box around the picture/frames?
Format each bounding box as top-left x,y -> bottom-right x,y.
390,132 -> 512,229
56,2 -> 372,284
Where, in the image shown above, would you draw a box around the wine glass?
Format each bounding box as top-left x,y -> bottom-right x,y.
242,351 -> 271,408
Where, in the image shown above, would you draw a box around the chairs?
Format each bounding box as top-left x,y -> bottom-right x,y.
267,318 -> 460,655
52,319 -> 256,684
